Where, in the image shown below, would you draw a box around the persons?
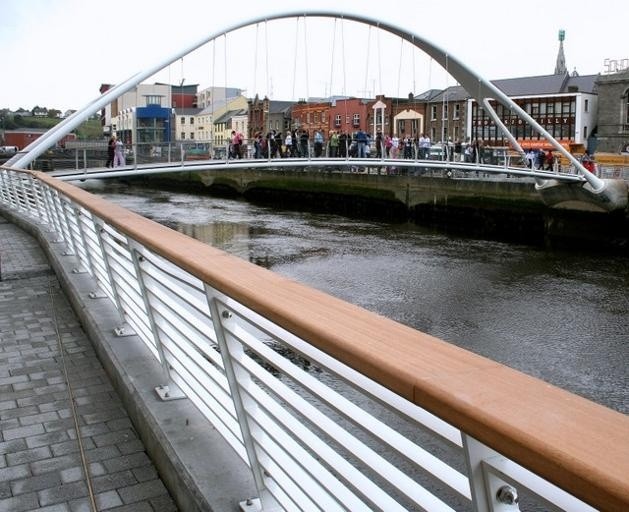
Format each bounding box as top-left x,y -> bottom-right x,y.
105,136 -> 116,167
113,139 -> 126,167
444,136 -> 485,164
230,131 -> 242,160
253,130 -> 430,161
525,148 -> 554,171
582,150 -> 598,173
